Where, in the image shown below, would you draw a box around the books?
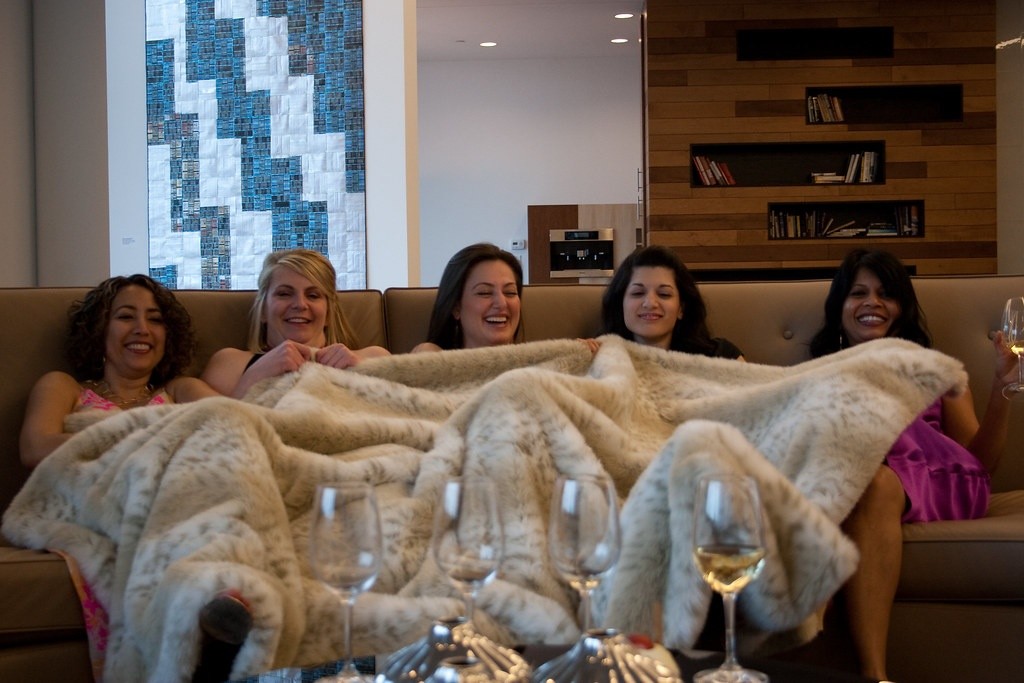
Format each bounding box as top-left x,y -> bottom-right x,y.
811,151 -> 883,184
807,93 -> 845,122
769,205 -> 919,238
692,155 -> 736,186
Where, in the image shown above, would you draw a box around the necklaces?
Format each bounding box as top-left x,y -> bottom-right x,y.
83,379 -> 154,409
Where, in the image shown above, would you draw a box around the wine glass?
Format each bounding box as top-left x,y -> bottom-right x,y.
1002,297 -> 1024,402
545,472 -> 618,632
692,475 -> 769,683
310,482 -> 383,683
433,475 -> 504,633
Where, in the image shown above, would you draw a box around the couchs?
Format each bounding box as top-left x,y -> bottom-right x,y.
0,275 -> 1023,682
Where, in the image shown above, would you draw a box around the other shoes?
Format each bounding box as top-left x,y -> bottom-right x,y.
201,594 -> 253,644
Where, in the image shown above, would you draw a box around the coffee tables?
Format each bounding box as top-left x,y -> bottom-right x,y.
243,641 -> 866,683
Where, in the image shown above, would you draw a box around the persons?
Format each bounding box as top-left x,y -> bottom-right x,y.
19,240 -> 1024,683
